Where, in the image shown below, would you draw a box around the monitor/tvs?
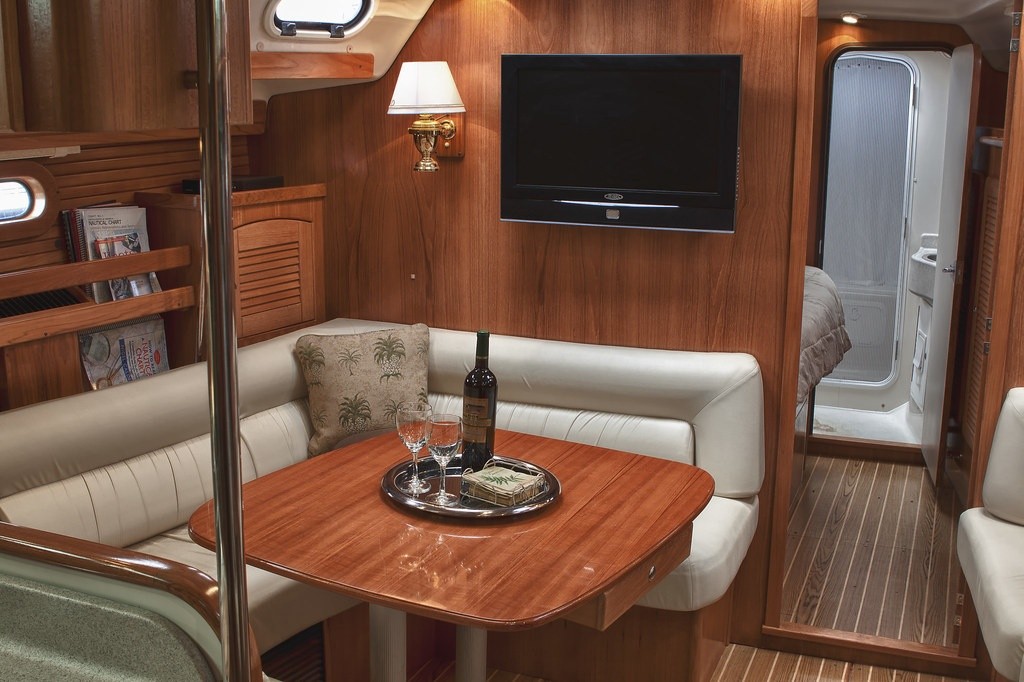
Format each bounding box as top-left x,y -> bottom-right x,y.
499,54 -> 742,232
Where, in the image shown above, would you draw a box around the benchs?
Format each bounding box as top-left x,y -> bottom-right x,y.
0,318 -> 765,682
957,387 -> 1024,682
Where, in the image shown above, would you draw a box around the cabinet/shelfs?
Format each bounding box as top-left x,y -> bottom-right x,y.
134,183 -> 330,365
16,0 -> 253,133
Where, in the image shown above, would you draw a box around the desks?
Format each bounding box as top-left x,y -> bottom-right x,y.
188,422 -> 715,682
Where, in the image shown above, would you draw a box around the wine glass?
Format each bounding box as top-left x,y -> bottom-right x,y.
396,402 -> 433,493
426,414 -> 459,506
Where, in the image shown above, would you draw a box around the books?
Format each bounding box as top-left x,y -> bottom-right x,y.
76,314 -> 170,391
59,200 -> 163,304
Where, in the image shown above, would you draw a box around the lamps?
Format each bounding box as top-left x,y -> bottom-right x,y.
387,61 -> 465,173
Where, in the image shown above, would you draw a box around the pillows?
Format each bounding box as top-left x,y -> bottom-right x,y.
295,323 -> 429,457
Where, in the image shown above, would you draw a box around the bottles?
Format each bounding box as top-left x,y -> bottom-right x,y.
462,329 -> 498,471
